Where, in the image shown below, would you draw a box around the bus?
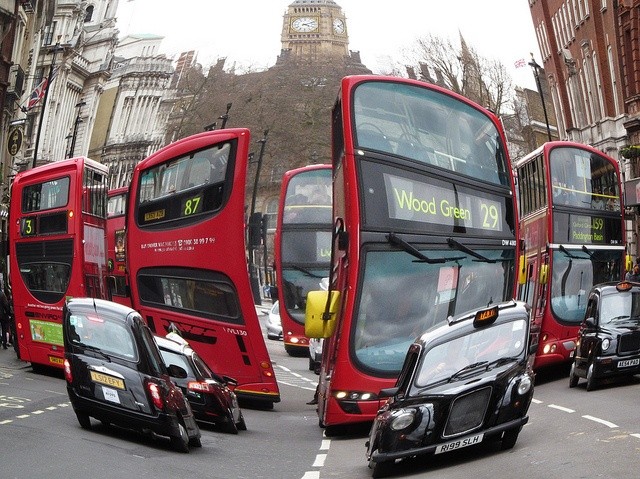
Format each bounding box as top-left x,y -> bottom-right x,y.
123,125 -> 281,408
316,74 -> 529,421
106,186 -> 131,307
273,163 -> 333,352
514,141 -> 632,370
9,157 -> 109,372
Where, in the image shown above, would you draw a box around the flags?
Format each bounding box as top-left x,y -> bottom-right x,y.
513,57 -> 527,69
26,65 -> 57,112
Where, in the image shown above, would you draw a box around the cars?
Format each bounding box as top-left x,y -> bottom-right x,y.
61,293 -> 204,453
365,298 -> 536,477
569,280 -> 640,390
152,332 -> 248,435
261,300 -> 283,339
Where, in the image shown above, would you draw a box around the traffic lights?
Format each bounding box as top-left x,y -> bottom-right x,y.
249,212 -> 265,246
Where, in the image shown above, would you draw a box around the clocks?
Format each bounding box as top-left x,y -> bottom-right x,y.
291,18 -> 318,33
333,18 -> 345,34
